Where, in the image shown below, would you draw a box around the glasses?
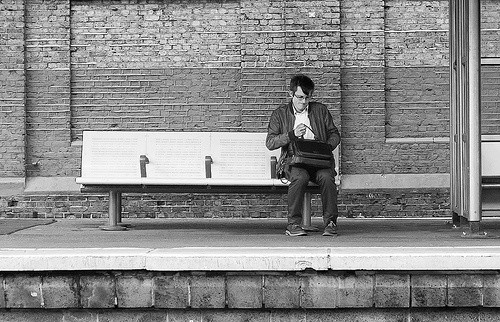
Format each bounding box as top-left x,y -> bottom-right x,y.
293,91 -> 313,101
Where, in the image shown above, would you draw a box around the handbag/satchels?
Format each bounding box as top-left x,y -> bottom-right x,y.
286,123 -> 335,171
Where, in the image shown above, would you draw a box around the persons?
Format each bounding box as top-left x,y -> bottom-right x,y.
266,75 -> 341,236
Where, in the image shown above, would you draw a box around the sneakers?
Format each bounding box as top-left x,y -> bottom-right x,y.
321,220 -> 338,237
284,222 -> 307,237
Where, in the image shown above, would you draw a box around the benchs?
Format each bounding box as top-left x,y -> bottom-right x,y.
76,130 -> 341,230
